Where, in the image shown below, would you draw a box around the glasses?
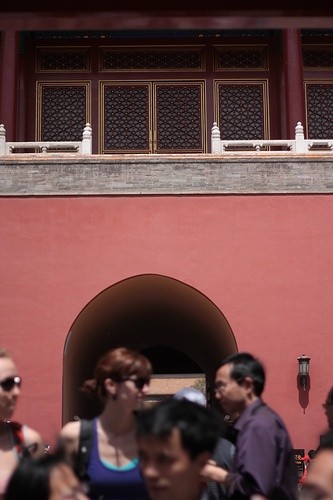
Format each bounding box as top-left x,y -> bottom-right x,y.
115,376 -> 147,389
0,375 -> 22,392
213,377 -> 244,392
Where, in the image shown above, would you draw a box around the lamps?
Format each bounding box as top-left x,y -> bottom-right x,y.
297,353 -> 311,391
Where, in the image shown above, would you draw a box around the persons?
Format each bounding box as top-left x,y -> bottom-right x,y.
199,353 -> 300,500
4,453 -> 90,500
135,400 -> 224,500
314,386 -> 333,453
55,346 -> 150,500
1,348 -> 47,500
